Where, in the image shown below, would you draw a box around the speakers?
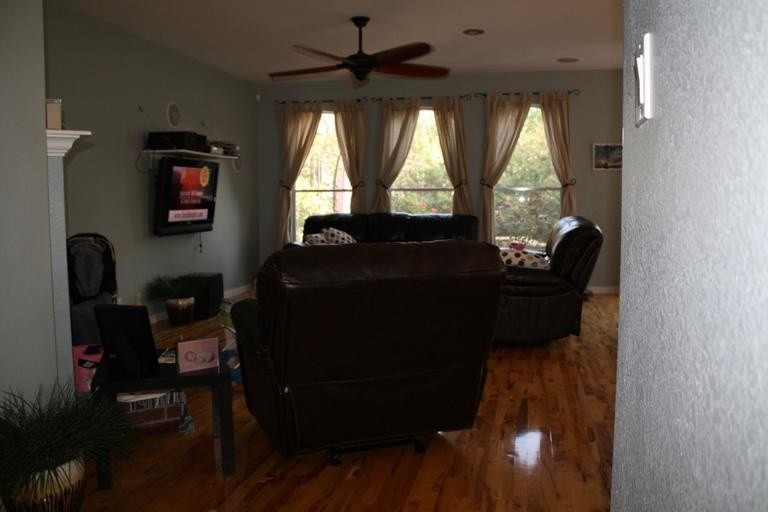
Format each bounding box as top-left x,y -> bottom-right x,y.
180,273 -> 223,320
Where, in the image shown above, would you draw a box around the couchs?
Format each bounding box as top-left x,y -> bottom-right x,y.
230,243 -> 507,460
304,213 -> 480,247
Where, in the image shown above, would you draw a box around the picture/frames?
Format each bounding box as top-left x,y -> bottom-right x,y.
592,142 -> 622,171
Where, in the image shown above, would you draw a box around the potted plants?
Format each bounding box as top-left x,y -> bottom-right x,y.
0,377 -> 146,512
144,272 -> 215,325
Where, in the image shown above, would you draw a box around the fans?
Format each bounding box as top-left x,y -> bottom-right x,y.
267,16 -> 450,82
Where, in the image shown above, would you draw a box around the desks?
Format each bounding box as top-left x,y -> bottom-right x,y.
91,348 -> 234,490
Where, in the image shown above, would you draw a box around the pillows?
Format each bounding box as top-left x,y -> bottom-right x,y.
499,246 -> 551,270
306,228 -> 357,245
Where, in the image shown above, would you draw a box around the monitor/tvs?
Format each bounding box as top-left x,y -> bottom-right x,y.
154,156 -> 221,236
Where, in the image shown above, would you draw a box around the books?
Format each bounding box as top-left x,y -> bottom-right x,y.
174,337 -> 220,376
158,346 -> 176,364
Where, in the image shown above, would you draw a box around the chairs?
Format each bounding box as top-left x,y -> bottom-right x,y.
494,214 -> 604,347
